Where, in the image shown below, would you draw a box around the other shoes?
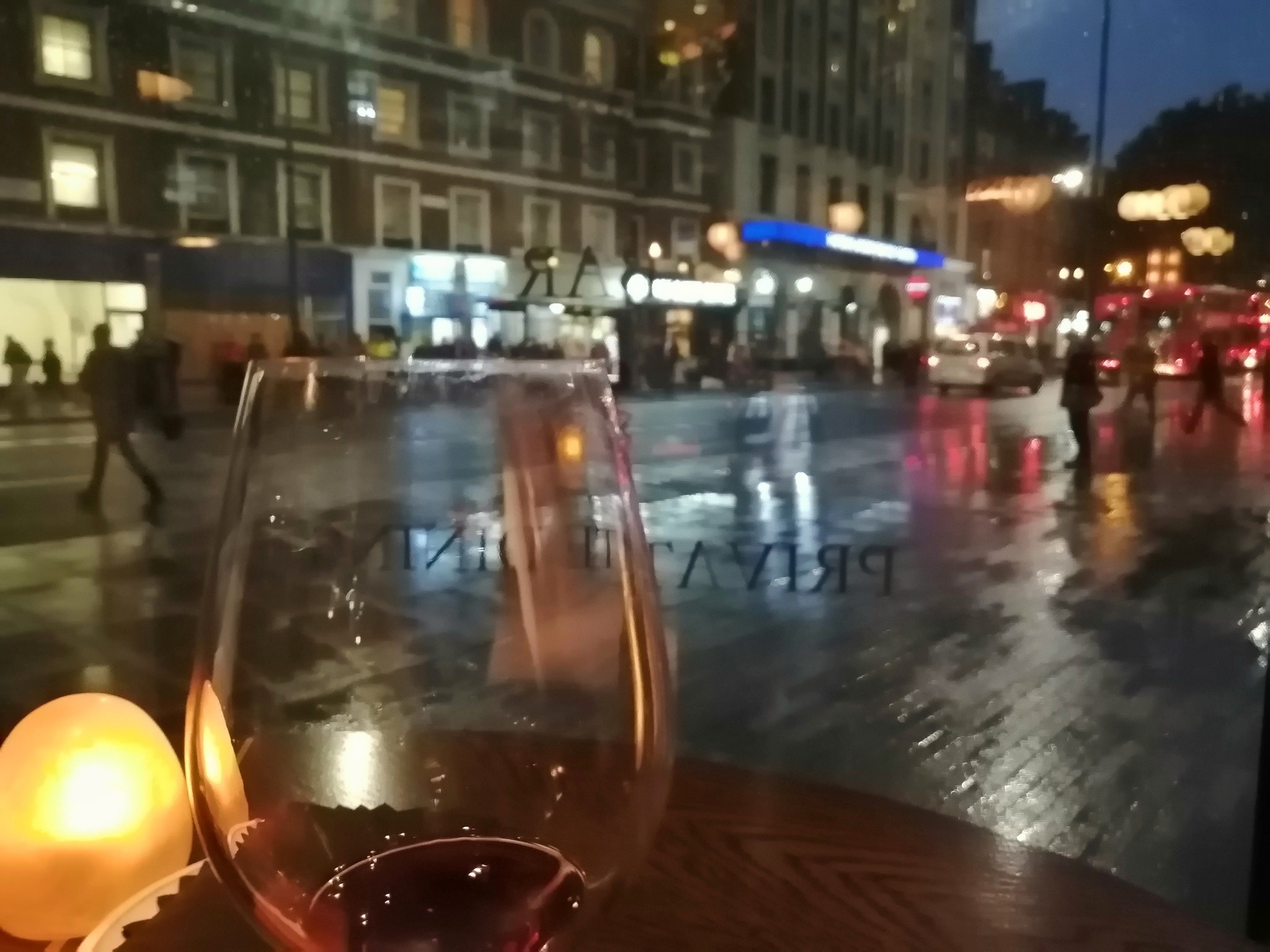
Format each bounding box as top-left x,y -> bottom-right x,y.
1065,454 -> 1094,470
140,491 -> 166,514
76,484 -> 100,507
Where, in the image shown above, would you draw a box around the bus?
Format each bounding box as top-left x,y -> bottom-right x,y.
1143,284 -> 1269,377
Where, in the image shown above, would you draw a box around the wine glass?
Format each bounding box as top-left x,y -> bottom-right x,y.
187,342 -> 678,952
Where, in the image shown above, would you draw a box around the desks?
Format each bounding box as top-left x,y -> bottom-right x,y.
1,717 -> 1257,952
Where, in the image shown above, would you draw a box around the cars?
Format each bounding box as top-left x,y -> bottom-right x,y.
926,333 -> 1045,397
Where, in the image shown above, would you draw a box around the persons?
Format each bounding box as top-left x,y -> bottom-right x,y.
1115,334 -> 1162,424
1059,324 -> 1104,478
73,323 -> 171,528
1,313 -> 1058,446
1181,333 -> 1251,432
